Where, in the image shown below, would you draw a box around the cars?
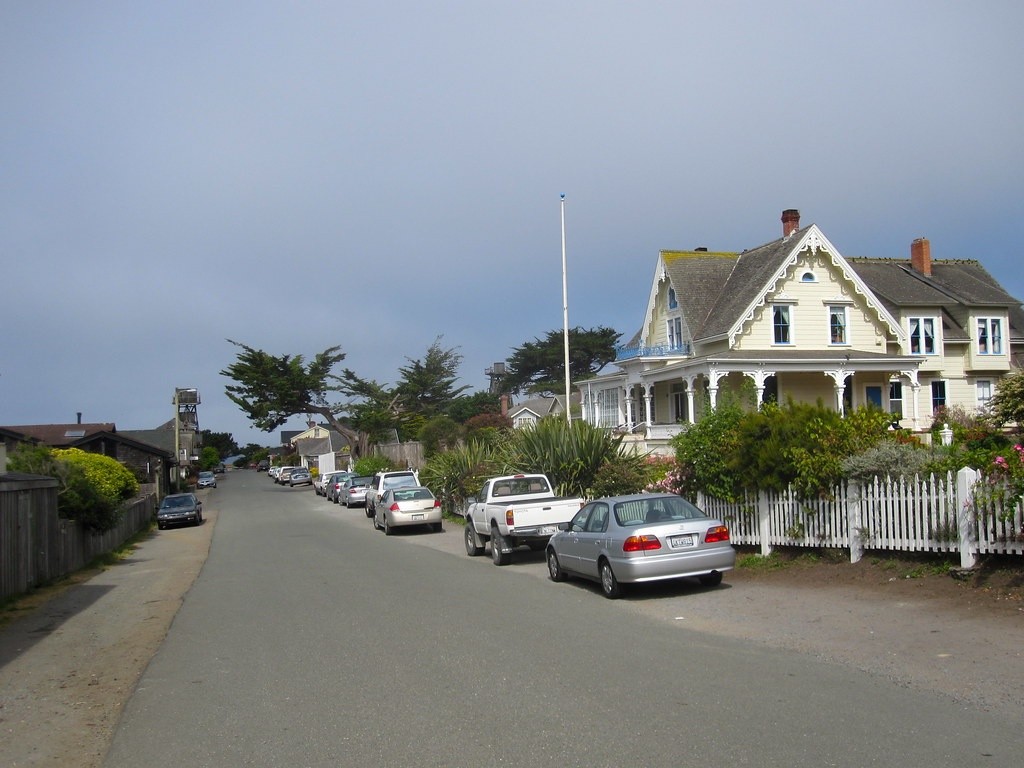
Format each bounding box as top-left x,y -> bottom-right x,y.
155,492 -> 203,530
374,485 -> 443,535
314,470 -> 348,497
256,459 -> 312,487
544,489 -> 737,599
197,471 -> 218,489
326,474 -> 360,504
338,476 -> 375,508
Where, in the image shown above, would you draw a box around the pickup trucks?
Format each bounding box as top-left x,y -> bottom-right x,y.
464,474 -> 586,566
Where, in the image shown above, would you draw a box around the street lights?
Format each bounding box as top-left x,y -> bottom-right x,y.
176,389 -> 197,494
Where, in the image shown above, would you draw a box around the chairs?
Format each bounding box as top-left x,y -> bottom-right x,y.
394,495 -> 401,501
645,509 -> 663,524
497,487 -> 512,496
414,492 -> 423,500
528,483 -> 542,493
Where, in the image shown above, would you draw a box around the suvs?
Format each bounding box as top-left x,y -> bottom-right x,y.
213,463 -> 224,474
364,471 -> 422,518
